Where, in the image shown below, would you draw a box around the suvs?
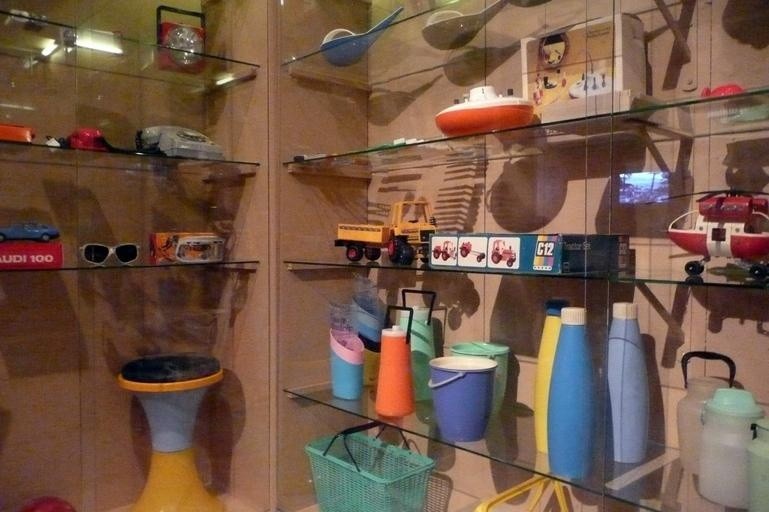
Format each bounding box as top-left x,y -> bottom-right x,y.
427,357 -> 497,443
696,388 -> 764,510
676,349 -> 737,474
450,341 -> 509,415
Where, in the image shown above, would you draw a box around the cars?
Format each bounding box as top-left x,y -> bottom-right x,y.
351,292 -> 389,386
429,354 -> 499,443
329,327 -> 366,401
449,342 -> 511,413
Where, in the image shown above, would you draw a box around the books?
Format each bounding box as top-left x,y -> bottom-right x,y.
305,422 -> 436,512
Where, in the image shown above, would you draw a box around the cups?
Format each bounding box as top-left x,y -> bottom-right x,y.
79,243 -> 139,266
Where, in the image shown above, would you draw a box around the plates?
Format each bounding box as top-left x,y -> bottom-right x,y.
277,0 -> 769,512
1,0 -> 270,512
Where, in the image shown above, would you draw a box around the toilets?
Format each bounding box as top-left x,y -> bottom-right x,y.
139,125 -> 227,162
67,126 -> 110,152
